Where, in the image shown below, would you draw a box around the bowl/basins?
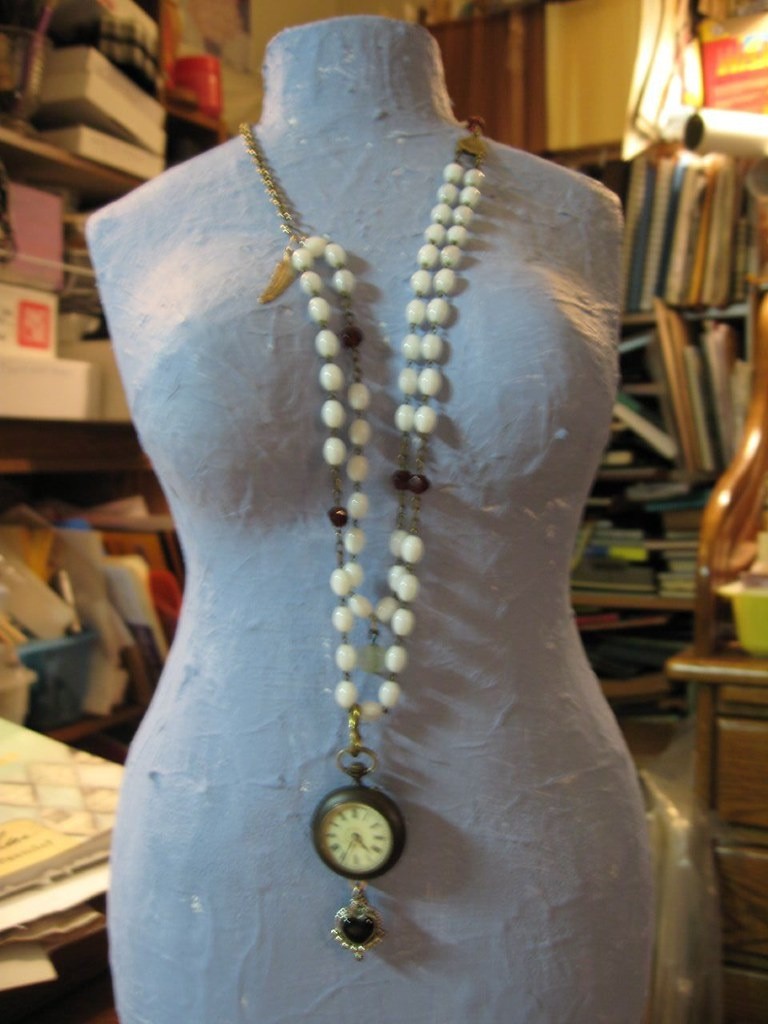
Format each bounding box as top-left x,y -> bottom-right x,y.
714,582 -> 768,658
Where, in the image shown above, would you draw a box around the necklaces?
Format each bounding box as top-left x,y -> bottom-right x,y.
217,122 -> 499,962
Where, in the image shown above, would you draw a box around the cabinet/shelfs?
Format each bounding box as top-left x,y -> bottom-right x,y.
540,137 -> 761,615
1,88 -> 228,741
664,644 -> 768,1024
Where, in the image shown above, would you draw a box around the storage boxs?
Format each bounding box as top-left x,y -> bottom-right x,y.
1,633 -> 101,731
0,50 -> 181,428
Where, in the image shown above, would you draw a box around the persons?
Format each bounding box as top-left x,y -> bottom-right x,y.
85,13 -> 656,1023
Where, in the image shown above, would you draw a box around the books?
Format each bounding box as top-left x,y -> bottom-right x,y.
585,144 -> 768,317
20,44 -> 170,180
570,604 -> 691,731
0,490 -> 192,712
595,293 -> 757,486
559,479 -> 715,601
